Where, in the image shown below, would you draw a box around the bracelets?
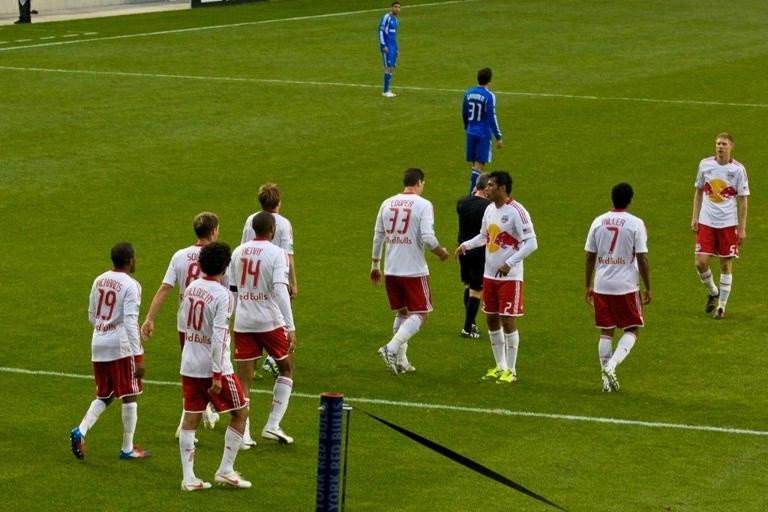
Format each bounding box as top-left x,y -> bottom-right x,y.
371,261 -> 381,273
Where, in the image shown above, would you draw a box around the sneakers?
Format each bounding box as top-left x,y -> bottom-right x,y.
377,343 -> 399,377
214,469 -> 252,490
705,287 -> 721,314
494,368 -> 519,385
252,370 -> 263,381
261,425 -> 294,445
601,362 -> 620,392
70,427 -> 86,461
239,438 -> 257,452
202,412 -> 219,431
381,89 -> 397,98
261,355 -> 280,379
481,366 -> 505,382
472,324 -> 479,333
460,328 -> 480,339
181,479 -> 213,492
602,382 -> 613,393
119,446 -> 150,459
713,306 -> 725,319
175,428 -> 200,444
397,360 -> 417,374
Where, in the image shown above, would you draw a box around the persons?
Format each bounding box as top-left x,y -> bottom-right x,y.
689,131 -> 750,320
70,242 -> 150,461
462,66 -> 503,197
139,211 -> 232,444
176,240 -> 253,493
370,168 -> 450,377
378,1 -> 402,98
582,182 -> 652,394
455,172 -> 491,340
227,211 -> 298,451
239,182 -> 299,379
453,170 -> 539,385
